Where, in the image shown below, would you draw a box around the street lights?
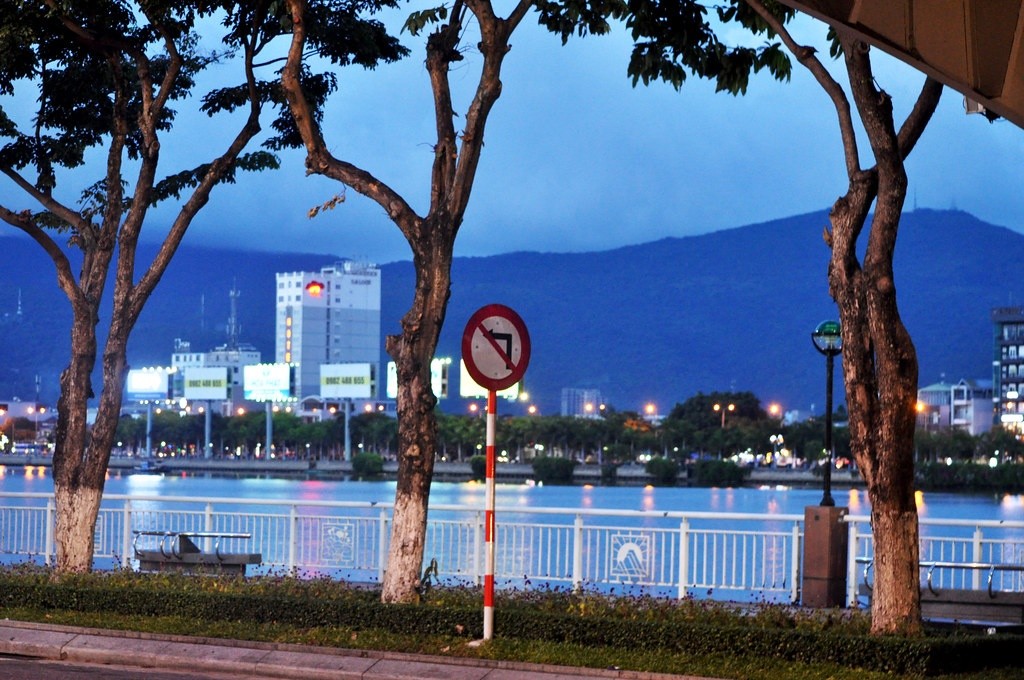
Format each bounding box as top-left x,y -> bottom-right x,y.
713,404 -> 735,460
28,406 -> 46,454
812,319 -> 846,505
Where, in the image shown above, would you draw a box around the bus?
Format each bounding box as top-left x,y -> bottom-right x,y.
15,443 -> 41,456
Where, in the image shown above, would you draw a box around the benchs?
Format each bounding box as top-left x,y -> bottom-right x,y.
132,530 -> 263,578
855,557 -> 1024,626
